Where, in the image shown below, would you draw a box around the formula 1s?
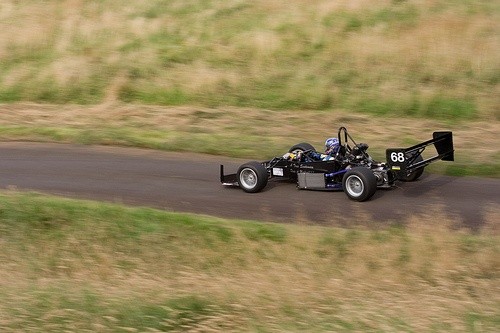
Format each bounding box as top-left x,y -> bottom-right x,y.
218,126 -> 456,202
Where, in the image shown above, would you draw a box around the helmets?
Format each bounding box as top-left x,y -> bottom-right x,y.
324,138 -> 341,154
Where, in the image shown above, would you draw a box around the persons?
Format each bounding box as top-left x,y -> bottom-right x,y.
287,137 -> 341,162
